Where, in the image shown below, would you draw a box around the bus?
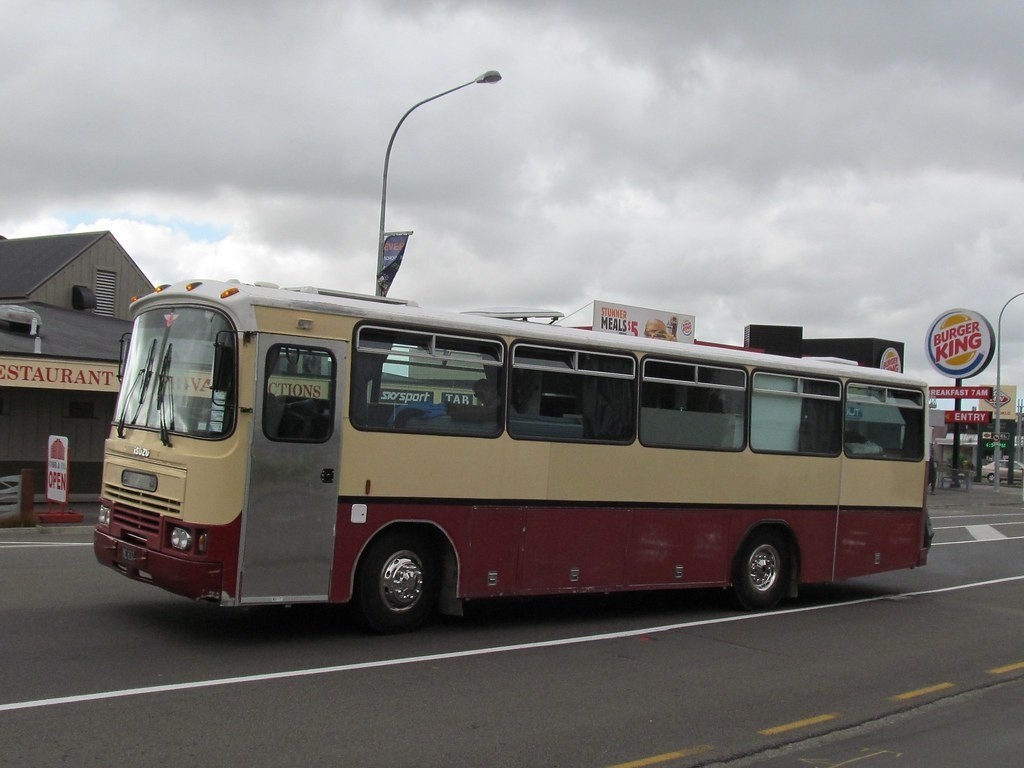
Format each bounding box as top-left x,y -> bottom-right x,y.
93,279 -> 935,633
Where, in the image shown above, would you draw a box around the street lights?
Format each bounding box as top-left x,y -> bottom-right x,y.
369,68 -> 502,405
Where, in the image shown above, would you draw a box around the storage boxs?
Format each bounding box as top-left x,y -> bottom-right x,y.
802,338 -> 904,373
743,324 -> 803,357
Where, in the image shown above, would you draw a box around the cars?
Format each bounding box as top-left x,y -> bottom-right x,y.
981,460 -> 1024,483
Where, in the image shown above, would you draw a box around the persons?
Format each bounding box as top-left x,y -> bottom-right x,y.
928,456 -> 937,496
472,378 -> 519,415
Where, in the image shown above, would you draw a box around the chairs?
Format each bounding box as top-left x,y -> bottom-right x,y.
512,369 -> 541,420
